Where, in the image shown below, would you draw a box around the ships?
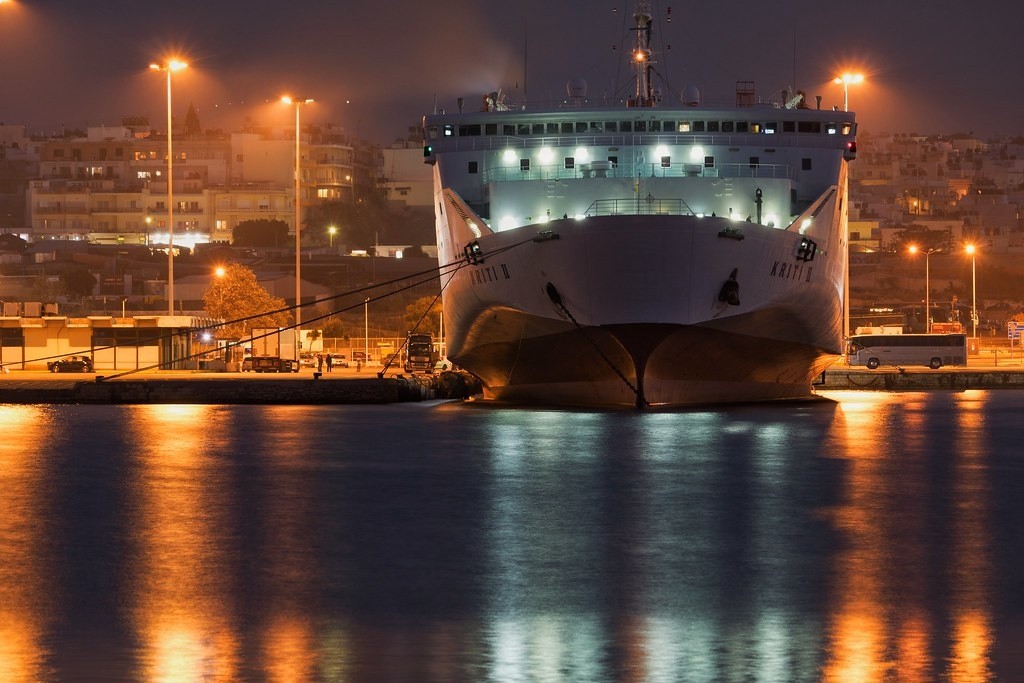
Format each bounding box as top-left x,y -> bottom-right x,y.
415,12 -> 861,407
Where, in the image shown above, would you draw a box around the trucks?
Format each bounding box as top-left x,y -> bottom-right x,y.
403,332 -> 439,376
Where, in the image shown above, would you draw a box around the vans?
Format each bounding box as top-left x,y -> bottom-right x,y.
244,356 -> 280,373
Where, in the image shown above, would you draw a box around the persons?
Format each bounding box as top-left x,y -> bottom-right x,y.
326,354 -> 332,372
317,354 -> 323,372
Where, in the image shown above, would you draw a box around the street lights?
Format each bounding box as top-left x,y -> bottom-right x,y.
909,245 -> 944,333
217,267 -> 225,318
965,243 -> 977,338
365,297 -> 371,367
280,94 -> 319,352
150,55 -> 189,307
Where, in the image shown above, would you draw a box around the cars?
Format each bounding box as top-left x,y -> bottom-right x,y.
351,352 -> 372,361
47,355 -> 94,374
300,353 -> 316,368
331,353 -> 348,368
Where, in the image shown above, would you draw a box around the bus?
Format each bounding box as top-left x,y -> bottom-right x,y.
842,333 -> 968,370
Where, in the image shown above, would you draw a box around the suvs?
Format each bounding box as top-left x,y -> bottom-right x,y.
385,354 -> 402,368
